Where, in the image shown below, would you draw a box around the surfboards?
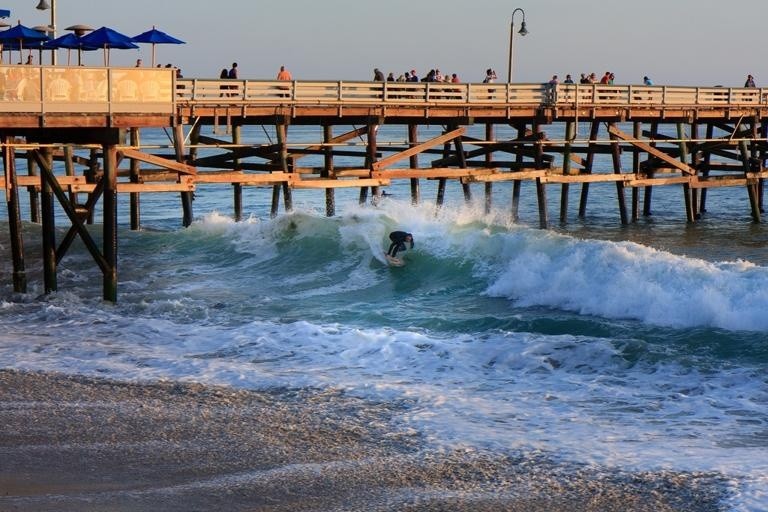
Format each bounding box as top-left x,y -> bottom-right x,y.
384,253 -> 402,266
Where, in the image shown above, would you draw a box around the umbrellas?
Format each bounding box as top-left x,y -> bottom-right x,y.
132,24 -> 186,67
0,16 -> 139,67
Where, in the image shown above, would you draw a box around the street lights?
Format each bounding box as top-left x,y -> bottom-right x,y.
36,0 -> 56,65
508,8 -> 530,83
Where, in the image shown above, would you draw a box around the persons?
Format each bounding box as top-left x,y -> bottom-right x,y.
549,70 -> 616,106
228,62 -> 238,106
164,64 -> 171,68
157,64 -> 161,68
384,229 -> 416,258
216,68 -> 232,106
372,67 -> 462,110
485,68 -> 497,109
134,57 -> 145,67
174,67 -> 185,97
743,74 -> 757,109
643,76 -> 655,108
277,64 -> 291,107
